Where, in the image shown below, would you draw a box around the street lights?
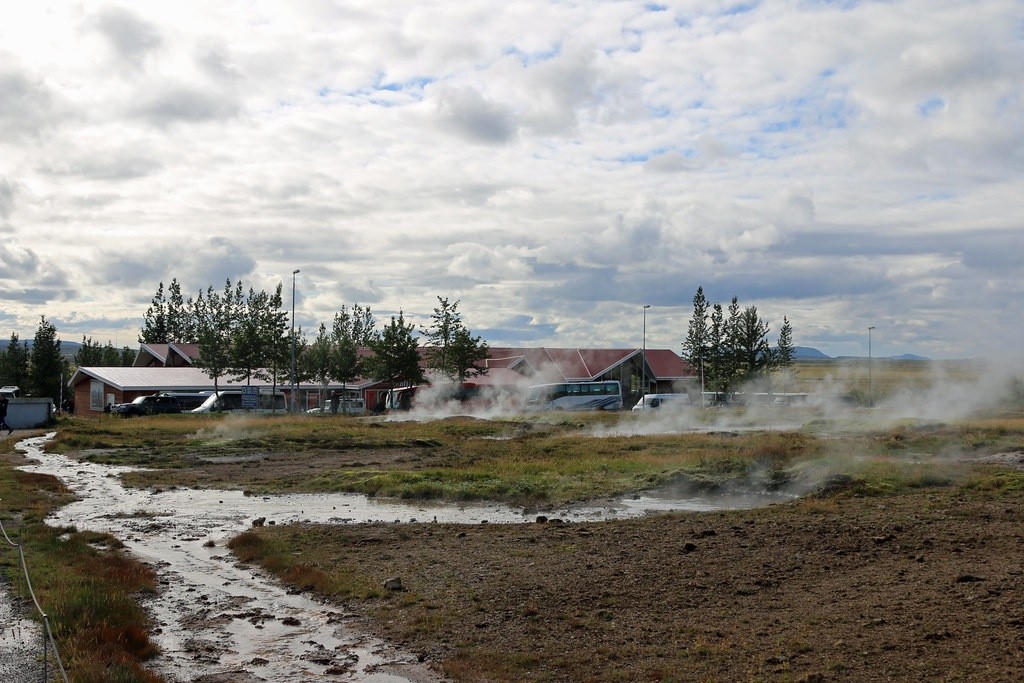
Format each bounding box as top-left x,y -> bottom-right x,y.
289,269 -> 301,415
641,304 -> 651,416
869,326 -> 876,407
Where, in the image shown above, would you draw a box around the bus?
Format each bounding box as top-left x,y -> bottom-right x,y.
190,390 -> 289,416
633,392 -> 859,410
157,390 -> 220,413
521,381 -> 624,414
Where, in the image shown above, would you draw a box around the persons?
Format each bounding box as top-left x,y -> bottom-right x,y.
103,403 -> 112,414
0,394 -> 13,435
61,398 -> 74,416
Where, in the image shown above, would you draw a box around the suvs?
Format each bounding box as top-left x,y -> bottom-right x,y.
111,395 -> 183,417
303,398 -> 367,415
